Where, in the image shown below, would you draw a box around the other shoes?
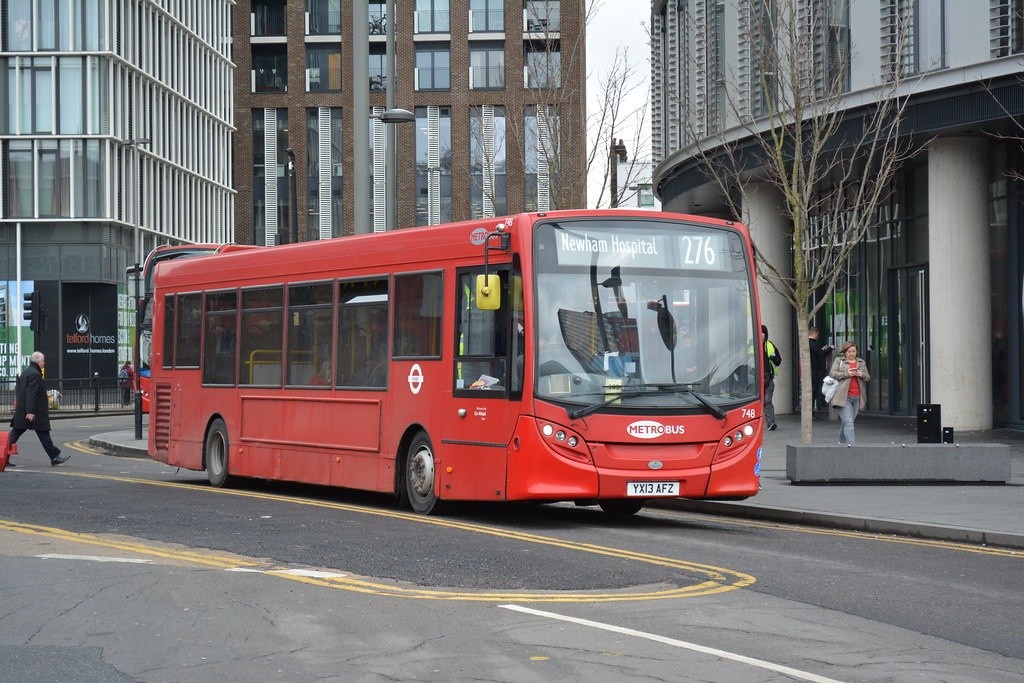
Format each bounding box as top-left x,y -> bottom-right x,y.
767,423 -> 777,430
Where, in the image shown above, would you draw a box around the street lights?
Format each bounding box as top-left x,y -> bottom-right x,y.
123,138 -> 152,439
353,108 -> 416,237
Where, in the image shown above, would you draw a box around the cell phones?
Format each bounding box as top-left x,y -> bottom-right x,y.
853,368 -> 858,371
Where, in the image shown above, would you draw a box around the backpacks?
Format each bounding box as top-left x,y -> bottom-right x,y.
119,368 -> 128,382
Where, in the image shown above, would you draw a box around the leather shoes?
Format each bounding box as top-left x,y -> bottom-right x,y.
51,455 -> 71,466
6,462 -> 15,467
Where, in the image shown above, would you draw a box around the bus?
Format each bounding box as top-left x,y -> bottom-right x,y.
146,209 -> 765,516
141,242 -> 265,413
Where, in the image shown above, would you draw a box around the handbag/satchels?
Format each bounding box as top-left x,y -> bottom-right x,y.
767,341 -> 782,366
822,376 -> 839,403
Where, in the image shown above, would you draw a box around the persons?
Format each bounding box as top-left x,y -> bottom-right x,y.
677,334 -> 697,384
120,361 -> 134,405
309,360 -> 331,386
747,323 -> 782,430
4,351 -> 72,466
822,341 -> 870,445
808,325 -> 835,420
